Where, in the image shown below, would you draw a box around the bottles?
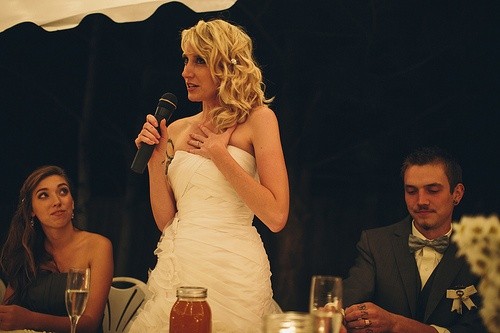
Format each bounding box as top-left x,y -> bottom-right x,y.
169,287 -> 213,333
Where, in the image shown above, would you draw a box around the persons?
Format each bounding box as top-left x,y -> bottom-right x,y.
315,147 -> 489,333
128,19 -> 290,333
0,166 -> 114,333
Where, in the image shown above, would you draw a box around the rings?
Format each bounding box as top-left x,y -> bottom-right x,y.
365,320 -> 369,325
197,141 -> 199,147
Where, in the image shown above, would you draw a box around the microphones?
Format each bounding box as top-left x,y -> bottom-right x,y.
131,94 -> 178,174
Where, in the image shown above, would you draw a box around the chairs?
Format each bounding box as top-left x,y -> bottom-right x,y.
102,276 -> 149,333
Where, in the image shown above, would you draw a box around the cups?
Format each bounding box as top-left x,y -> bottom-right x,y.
309,276 -> 343,333
266,314 -> 312,333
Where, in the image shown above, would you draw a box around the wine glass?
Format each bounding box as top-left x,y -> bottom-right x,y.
65,267 -> 90,333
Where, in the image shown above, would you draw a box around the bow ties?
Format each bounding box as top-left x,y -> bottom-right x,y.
408,234 -> 449,253
